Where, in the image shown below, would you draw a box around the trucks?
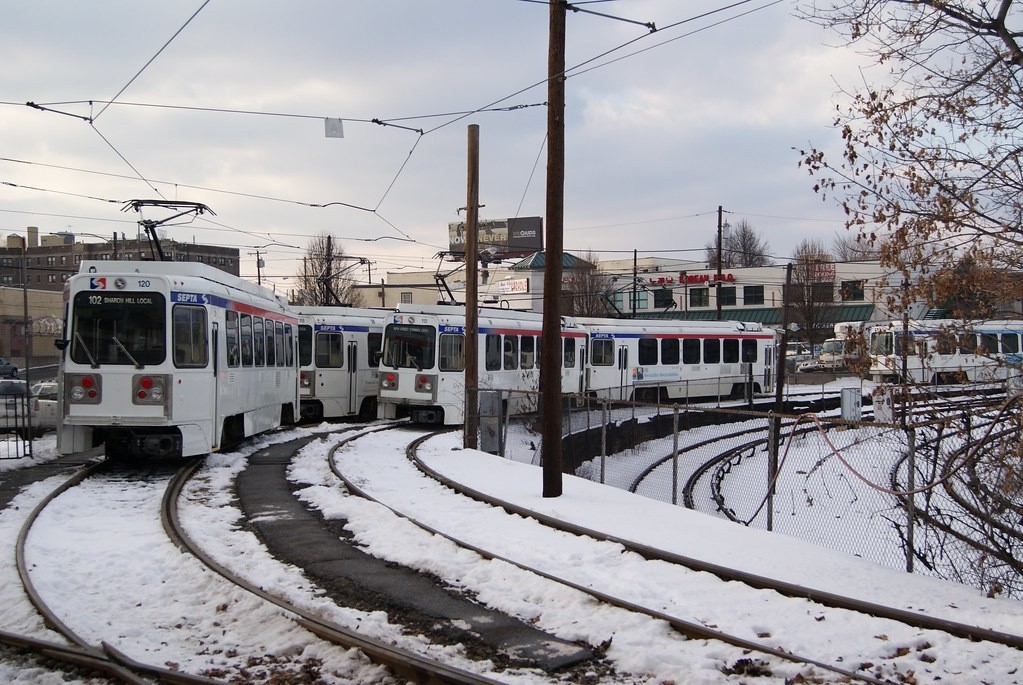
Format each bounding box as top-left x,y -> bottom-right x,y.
819,320 -> 891,373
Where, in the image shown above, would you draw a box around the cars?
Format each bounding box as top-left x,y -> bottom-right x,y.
20,382 -> 57,438
798,359 -> 819,372
776,357 -> 796,374
0,357 -> 18,378
0,380 -> 39,442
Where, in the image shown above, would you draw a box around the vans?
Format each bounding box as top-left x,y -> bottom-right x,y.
778,341 -> 822,371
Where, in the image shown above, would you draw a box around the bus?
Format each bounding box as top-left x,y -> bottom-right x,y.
372,302 -> 589,426
866,318 -> 1023,386
289,302 -> 777,424
53,258 -> 299,464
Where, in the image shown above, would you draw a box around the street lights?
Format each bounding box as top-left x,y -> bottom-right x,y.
707,282 -> 721,320
